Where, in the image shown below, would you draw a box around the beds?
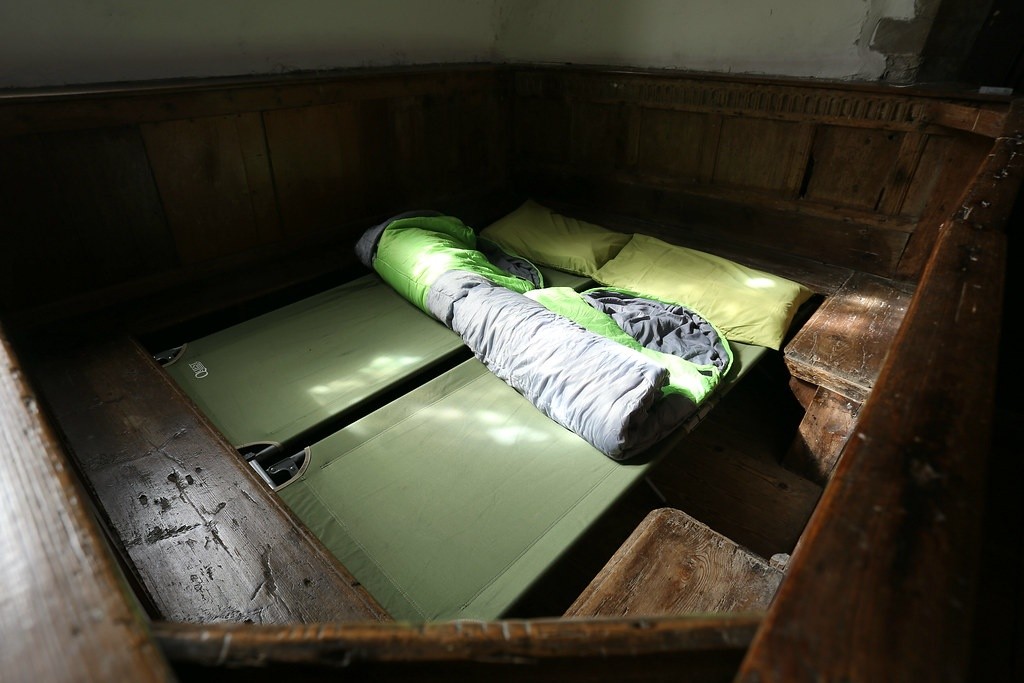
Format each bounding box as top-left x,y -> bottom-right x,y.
152,196 -> 818,620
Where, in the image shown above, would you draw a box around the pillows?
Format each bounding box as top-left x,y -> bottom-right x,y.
594,231 -> 816,351
480,197 -> 635,277
